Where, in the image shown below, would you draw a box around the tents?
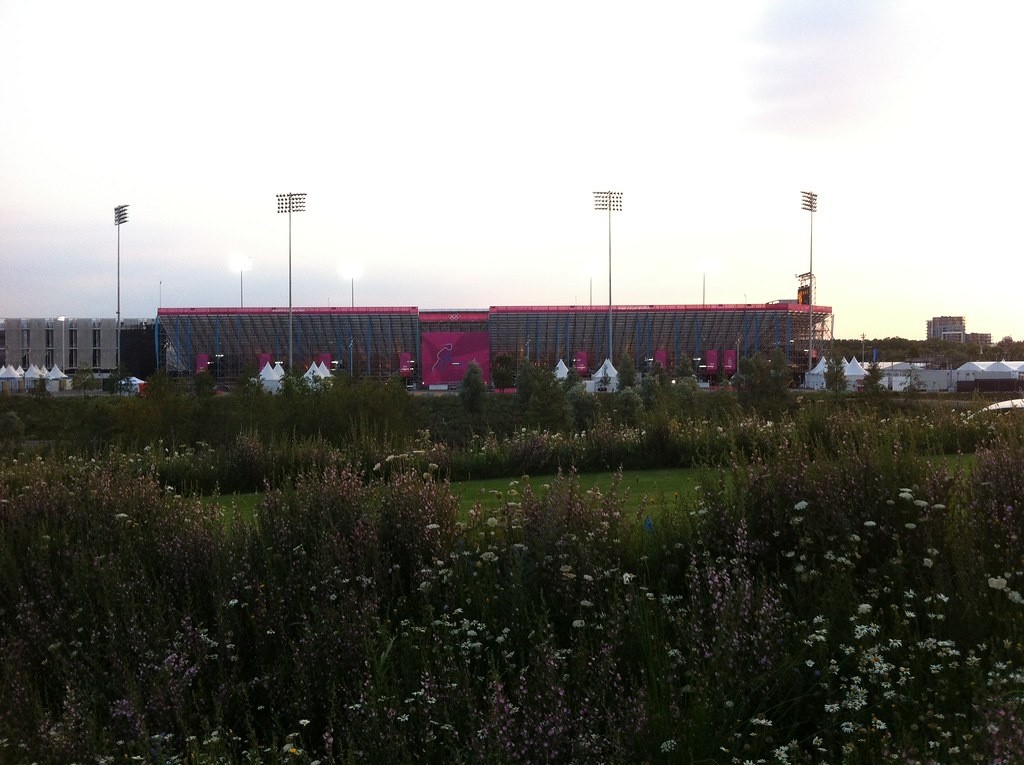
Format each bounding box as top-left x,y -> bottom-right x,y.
0,364 -> 72,395
115,376 -> 150,399
803,359 -> 1024,396
551,357 -> 620,394
257,360 -> 337,397
